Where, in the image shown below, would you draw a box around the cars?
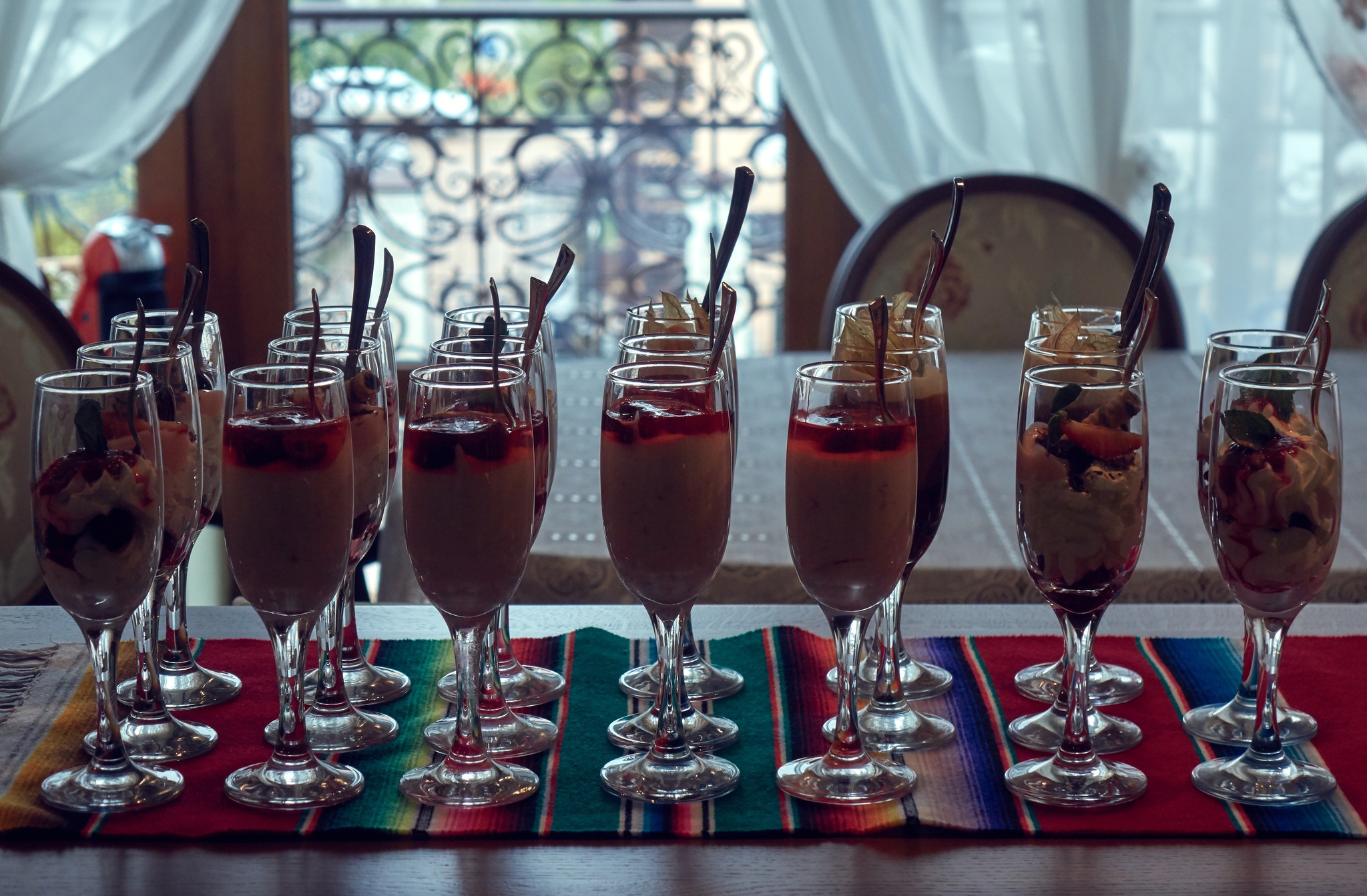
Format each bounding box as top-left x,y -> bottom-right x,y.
306,71 -> 473,128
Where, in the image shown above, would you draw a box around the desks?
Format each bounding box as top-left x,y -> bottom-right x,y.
371,345 -> 1367,601
0,581 -> 1367,896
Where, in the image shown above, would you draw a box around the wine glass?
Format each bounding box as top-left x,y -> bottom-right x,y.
398,362 -> 539,812
1181,327 -> 1320,748
30,367 -> 185,814
280,305 -> 411,707
263,334 -> 400,754
434,303 -> 567,709
75,336 -> 220,766
826,300 -> 954,700
604,332 -> 741,756
1004,362 -> 1151,812
222,361 -> 366,812
109,308 -> 243,713
600,361 -> 741,805
1191,366 -> 1344,806
1008,328 -> 1144,757
822,333 -> 957,759
617,299 -> 744,702
1015,307 -> 1146,707
423,336 -> 561,765
775,362 -> 919,808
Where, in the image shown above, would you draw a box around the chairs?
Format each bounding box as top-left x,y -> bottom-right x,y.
810,169 -> 1190,351
1285,189 -> 1367,349
0,261 -> 102,605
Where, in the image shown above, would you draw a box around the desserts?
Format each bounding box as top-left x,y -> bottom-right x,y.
35,343 -> 228,618
1009,352 -> 1340,614
